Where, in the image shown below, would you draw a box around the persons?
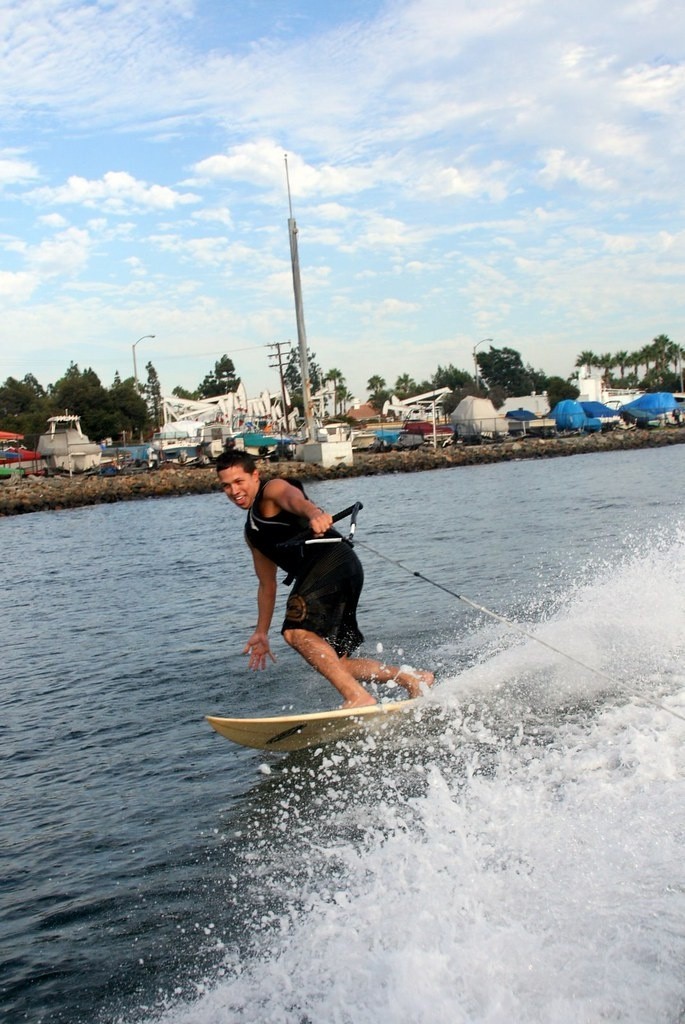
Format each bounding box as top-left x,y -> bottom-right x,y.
215,449 -> 435,709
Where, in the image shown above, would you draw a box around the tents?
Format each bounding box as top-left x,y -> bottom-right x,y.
618,391 -> 685,428
374,394 -> 620,445
0,431 -> 42,477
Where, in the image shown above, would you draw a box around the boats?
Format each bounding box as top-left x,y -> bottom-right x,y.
0,389 -> 685,475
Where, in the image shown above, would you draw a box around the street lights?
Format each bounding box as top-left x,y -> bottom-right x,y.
131,335 -> 156,394
473,337 -> 494,390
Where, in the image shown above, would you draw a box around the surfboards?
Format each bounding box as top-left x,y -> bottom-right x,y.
204,696 -> 421,752
